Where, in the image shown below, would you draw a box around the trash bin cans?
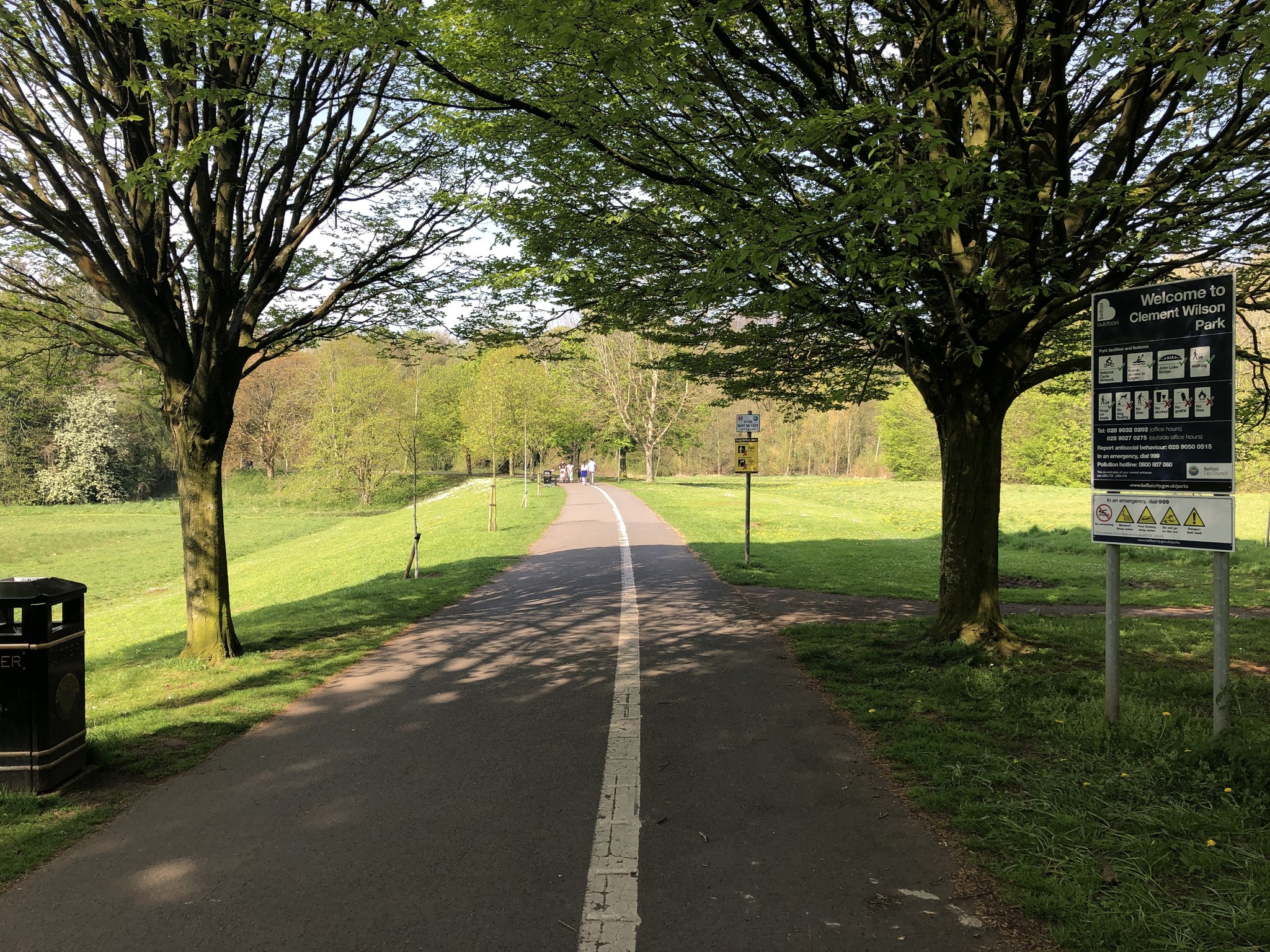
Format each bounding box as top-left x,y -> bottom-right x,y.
544,470 -> 551,484
0,577 -> 86,795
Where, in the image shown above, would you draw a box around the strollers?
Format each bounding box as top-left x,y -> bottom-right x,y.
559,468 -> 567,483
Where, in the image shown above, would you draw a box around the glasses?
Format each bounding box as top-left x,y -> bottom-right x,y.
569,463 -> 571,464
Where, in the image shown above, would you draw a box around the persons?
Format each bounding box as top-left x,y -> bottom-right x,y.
575,466 -> 582,482
588,458 -> 597,486
558,459 -> 567,482
581,461 -> 588,486
567,461 -> 575,483
